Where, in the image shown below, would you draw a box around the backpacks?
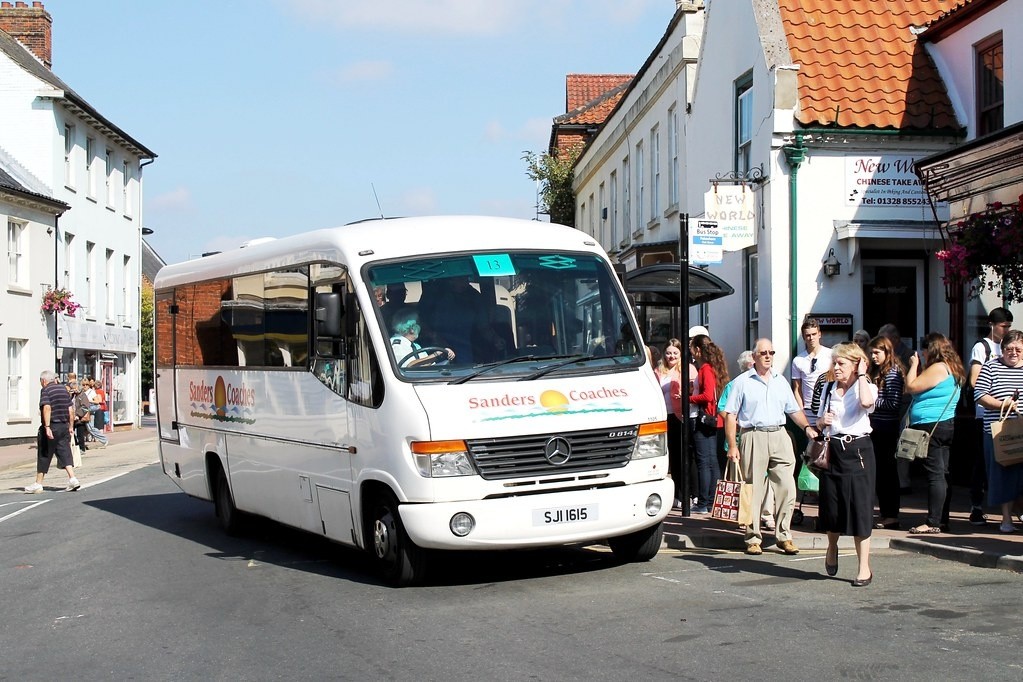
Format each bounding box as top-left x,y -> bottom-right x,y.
955,338 -> 992,423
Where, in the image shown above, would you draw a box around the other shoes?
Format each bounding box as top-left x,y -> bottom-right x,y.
825,545 -> 838,576
1000,520 -> 1014,532
969,517 -> 985,525
747,544 -> 762,555
690,505 -> 708,515
852,572 -> 872,586
909,526 -> 941,533
872,520 -> 902,528
776,539 -> 799,554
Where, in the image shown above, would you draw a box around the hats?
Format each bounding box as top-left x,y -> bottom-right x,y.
689,326 -> 709,337
387,283 -> 406,292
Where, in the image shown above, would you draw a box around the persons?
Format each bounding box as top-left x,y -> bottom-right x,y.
436,276 -> 506,367
379,282 -> 412,312
620,325 -> 729,515
61,372 -> 109,451
23,370 -> 81,493
812,341 -> 883,588
389,306 -> 455,367
966,305 -> 1023,531
714,318 -> 921,556
902,332 -> 966,534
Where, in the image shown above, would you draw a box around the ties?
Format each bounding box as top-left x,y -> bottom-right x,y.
411,344 -> 419,360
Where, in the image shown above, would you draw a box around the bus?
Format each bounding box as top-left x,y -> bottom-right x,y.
154,214 -> 676,590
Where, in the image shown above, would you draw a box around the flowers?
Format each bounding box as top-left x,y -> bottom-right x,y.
42,284 -> 85,319
933,188 -> 1023,305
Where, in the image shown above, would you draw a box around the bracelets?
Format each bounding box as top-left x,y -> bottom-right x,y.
858,373 -> 865,377
804,425 -> 810,431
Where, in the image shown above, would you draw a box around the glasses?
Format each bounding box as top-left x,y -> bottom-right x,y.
760,351 -> 776,355
83,383 -> 90,386
811,359 -> 817,372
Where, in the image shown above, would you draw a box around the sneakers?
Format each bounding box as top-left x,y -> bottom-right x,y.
65,480 -> 81,491
25,483 -> 43,494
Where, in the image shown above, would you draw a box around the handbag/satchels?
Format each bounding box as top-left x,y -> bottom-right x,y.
894,428 -> 930,461
695,408 -> 717,437
104,412 -> 111,425
710,458 -> 752,526
89,403 -> 101,411
57,435 -> 82,469
991,398 -> 1023,467
800,441 -> 829,472
798,463 -> 819,492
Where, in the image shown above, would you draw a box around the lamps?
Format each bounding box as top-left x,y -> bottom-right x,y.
823,248 -> 843,278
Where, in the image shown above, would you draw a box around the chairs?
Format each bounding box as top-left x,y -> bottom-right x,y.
383,302 -> 449,362
